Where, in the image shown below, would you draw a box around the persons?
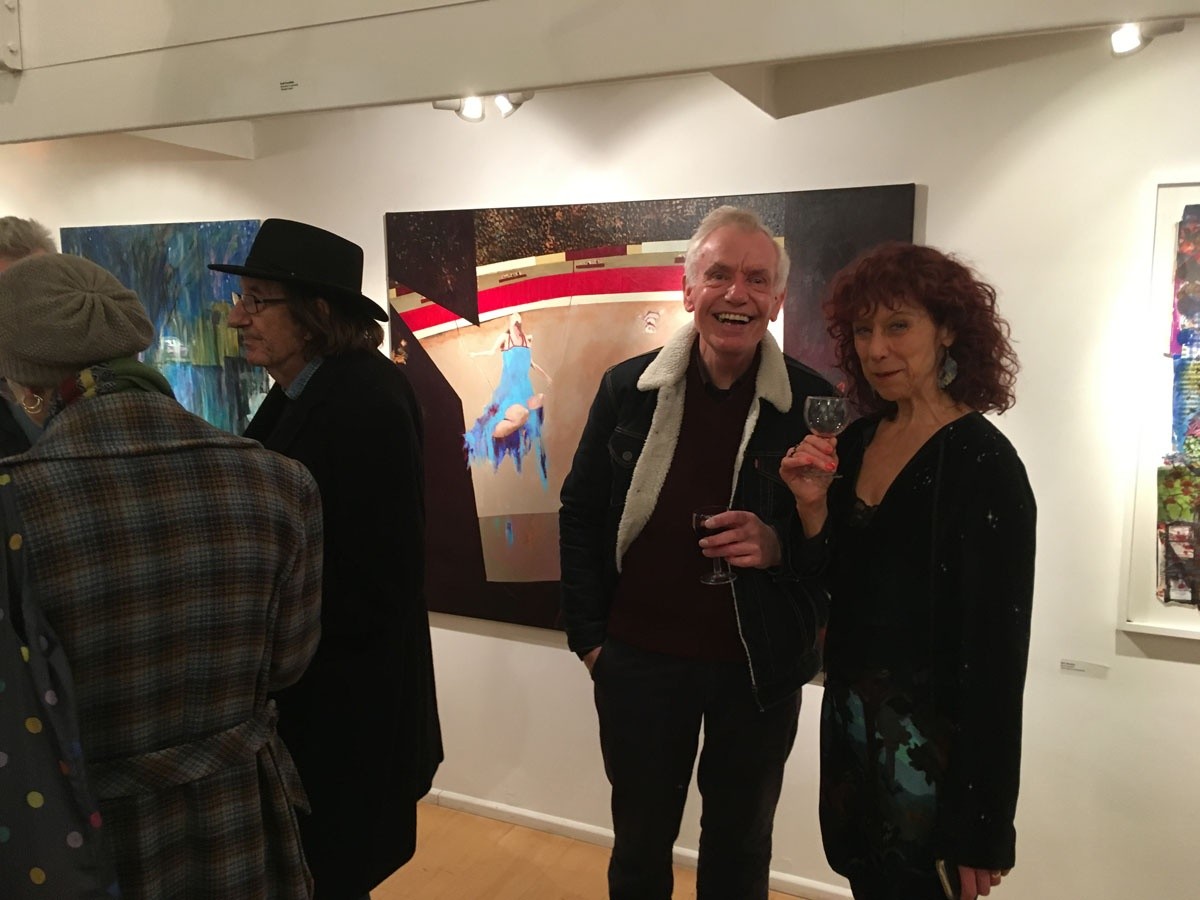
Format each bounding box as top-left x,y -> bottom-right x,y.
779,243 -> 1037,900
559,206 -> 843,900
208,218 -> 445,900
0,216 -> 322,900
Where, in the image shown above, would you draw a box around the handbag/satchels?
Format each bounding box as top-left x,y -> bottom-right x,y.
0,466 -> 111,900
821,678 -> 960,874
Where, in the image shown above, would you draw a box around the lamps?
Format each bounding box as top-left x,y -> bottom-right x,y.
431,96 -> 485,122
1111,15 -> 1183,58
495,89 -> 534,117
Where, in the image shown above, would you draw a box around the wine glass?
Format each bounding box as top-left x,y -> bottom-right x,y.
690,506 -> 737,585
804,395 -> 853,480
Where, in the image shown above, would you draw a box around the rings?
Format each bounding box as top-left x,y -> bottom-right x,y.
788,451 -> 796,458
991,872 -> 1002,878
792,444 -> 801,451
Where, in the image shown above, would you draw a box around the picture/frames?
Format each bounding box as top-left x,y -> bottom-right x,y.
1116,179 -> 1200,640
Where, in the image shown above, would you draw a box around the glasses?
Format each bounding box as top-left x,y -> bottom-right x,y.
232,291 -> 288,314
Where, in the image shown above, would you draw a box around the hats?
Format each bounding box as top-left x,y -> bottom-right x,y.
0,253 -> 154,388
207,219 -> 389,322
